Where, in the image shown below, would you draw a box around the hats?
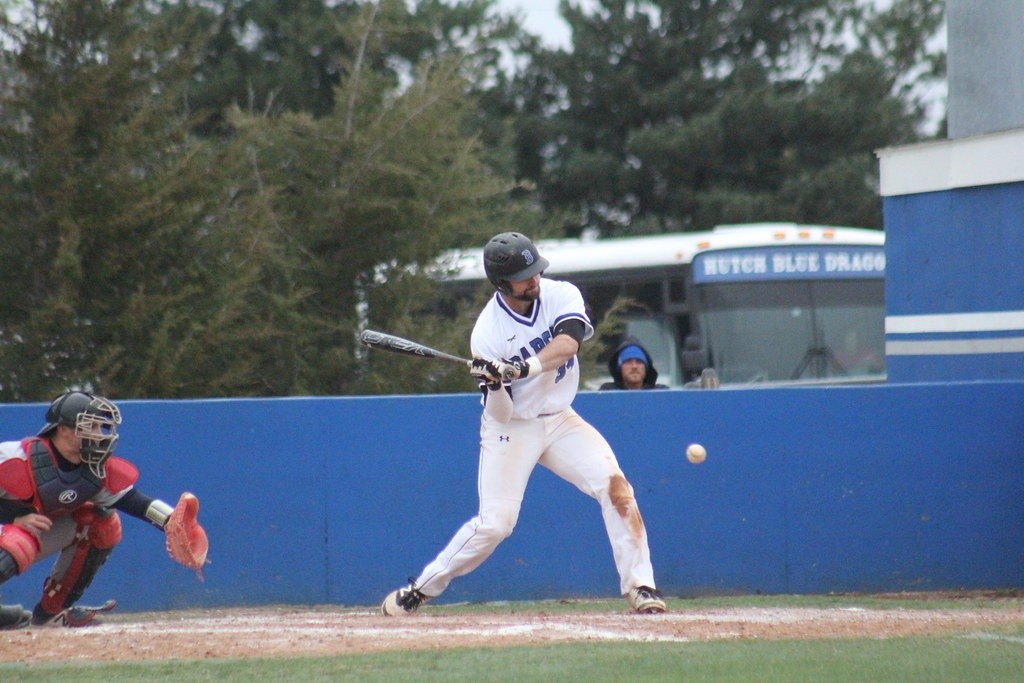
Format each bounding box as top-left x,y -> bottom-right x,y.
34,390 -> 102,437
617,346 -> 648,366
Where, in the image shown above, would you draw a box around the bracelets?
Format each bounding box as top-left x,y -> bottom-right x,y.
526,357 -> 541,377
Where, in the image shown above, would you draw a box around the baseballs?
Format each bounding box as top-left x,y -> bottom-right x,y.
687,444 -> 707,465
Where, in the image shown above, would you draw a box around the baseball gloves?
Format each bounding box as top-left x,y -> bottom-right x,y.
164,491 -> 208,569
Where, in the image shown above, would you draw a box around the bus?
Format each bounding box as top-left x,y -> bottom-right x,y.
351,220 -> 886,383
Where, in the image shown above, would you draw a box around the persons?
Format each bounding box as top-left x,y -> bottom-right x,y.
597,336 -> 670,390
380,231 -> 667,617
0,391 -> 208,630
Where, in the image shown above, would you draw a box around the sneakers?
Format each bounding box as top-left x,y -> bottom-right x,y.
31,600 -> 117,628
626,585 -> 666,614
381,576 -> 428,618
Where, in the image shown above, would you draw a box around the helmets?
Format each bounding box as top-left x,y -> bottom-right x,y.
483,232 -> 550,294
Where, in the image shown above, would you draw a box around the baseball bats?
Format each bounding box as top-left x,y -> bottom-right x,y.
360,329 -> 514,380
